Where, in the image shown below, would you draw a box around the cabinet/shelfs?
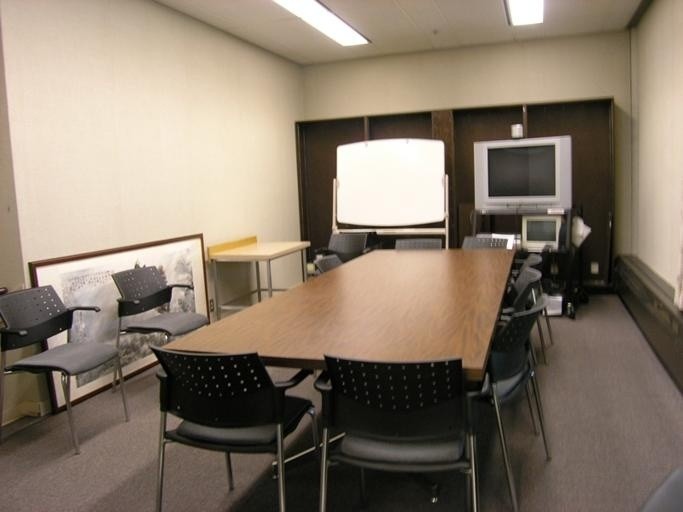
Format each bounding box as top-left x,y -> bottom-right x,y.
472,205 -> 588,319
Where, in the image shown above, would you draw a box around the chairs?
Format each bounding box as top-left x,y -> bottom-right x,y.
461,237 -> 509,249
149,342 -> 322,511
314,232 -> 377,258
395,239 -> 442,250
313,254 -> 342,272
482,252 -> 551,512
313,354 -> 489,512
1,285 -> 132,456
111,266 -> 210,394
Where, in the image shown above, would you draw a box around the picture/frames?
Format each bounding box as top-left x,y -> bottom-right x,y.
27,232 -> 212,415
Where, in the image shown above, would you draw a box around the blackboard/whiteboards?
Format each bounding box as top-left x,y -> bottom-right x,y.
336,138 -> 445,226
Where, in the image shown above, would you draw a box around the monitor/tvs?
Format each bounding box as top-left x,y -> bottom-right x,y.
521,215 -> 567,253
473,134 -> 573,216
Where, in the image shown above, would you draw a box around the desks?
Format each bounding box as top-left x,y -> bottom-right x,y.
160,246 -> 519,381
207,237 -> 313,323
